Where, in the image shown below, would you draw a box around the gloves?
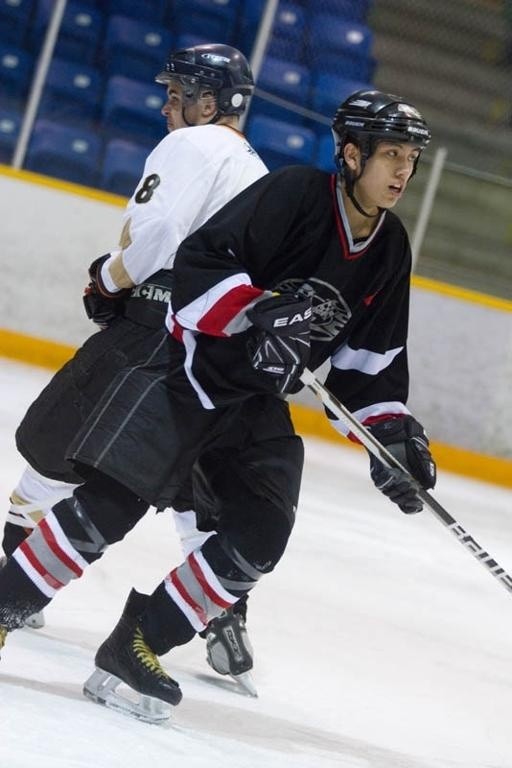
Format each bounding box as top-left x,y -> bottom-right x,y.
245,293 -> 312,394
363,415 -> 436,515
82,253 -> 128,330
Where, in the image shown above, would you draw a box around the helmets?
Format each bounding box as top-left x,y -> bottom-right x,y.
154,43 -> 254,115
330,89 -> 432,169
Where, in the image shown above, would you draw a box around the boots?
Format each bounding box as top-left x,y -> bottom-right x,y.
206,612 -> 254,675
95,587 -> 183,706
0,555 -> 45,652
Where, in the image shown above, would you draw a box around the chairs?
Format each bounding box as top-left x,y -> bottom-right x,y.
1,2 -> 381,199
